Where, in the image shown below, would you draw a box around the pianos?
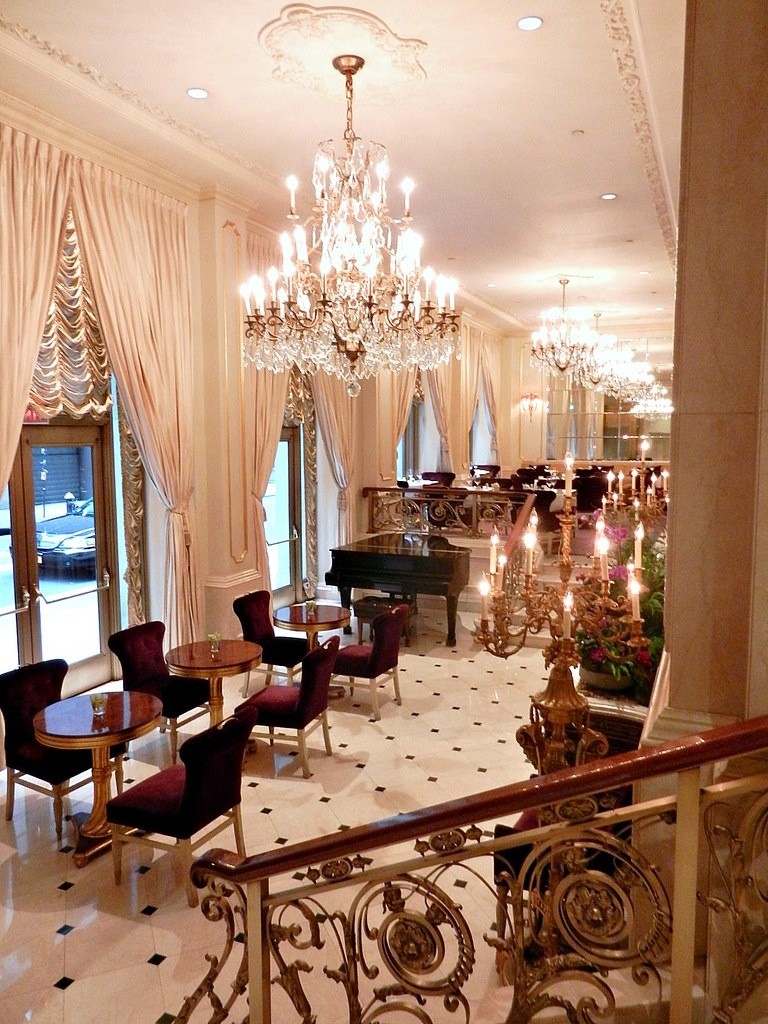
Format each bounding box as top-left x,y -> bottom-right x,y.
325,533 -> 474,648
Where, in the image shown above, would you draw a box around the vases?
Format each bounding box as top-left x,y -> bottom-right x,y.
580,664 -> 633,692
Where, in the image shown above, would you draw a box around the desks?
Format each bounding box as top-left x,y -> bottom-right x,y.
272,605 -> 351,698
165,639 -> 264,764
32,693 -> 164,865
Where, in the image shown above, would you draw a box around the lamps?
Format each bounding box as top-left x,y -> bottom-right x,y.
474,467 -> 669,662
238,53 -> 465,396
531,279 -> 673,420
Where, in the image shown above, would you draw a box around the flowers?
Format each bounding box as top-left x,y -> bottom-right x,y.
576,512 -> 668,686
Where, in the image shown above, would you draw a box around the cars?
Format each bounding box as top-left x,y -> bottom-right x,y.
9,497 -> 96,569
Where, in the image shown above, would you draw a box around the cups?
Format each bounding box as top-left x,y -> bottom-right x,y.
90,694 -> 108,714
207,634 -> 222,652
306,601 -> 315,614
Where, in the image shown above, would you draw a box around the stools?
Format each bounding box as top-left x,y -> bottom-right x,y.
354,596 -> 411,648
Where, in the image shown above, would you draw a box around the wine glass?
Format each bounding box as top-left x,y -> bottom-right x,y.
416,471 -> 423,482
407,469 -> 414,482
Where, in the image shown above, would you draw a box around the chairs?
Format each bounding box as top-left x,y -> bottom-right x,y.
109,620 -> 211,766
0,658 -> 127,843
233,590 -> 319,698
495,775 -> 632,968
233,635 -> 341,779
104,704 -> 258,907
333,603 -> 410,722
